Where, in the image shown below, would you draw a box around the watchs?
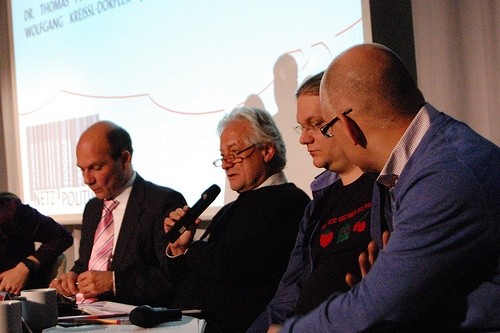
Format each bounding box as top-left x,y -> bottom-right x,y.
22,258 -> 35,273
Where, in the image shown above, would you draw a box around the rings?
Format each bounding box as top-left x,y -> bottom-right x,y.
54,278 -> 58,281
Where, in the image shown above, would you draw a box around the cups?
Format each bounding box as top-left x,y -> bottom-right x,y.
13,288 -> 58,329
0,300 -> 23,333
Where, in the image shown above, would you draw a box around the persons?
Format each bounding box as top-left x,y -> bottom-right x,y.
266,43 -> 500,333
164,104 -> 311,333
49,121 -> 189,309
0,192 -> 74,301
248,71 -> 378,333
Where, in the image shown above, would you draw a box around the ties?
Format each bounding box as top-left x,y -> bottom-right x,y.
75,200 -> 120,305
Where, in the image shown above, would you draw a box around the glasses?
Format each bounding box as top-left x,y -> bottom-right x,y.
213,142 -> 262,167
293,120 -> 323,134
320,108 -> 352,138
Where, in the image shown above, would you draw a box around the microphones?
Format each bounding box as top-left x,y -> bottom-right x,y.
130,307 -> 180,328
163,184 -> 221,243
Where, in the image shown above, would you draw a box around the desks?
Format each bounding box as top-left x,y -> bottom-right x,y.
43,301 -> 206,333
